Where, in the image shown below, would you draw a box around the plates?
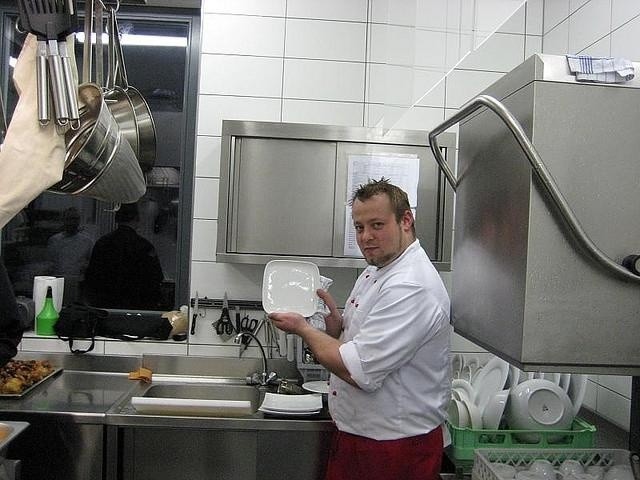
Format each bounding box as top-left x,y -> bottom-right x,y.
262,259 -> 321,318
450,354 -> 588,444
257,380 -> 330,416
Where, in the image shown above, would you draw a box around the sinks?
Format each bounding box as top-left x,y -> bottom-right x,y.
117,378 -> 266,419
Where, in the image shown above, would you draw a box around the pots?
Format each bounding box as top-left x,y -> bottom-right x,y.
48,0 -> 158,193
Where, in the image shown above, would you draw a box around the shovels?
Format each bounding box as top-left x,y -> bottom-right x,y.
24,0 -> 71,127
18,0 -> 51,128
57,0 -> 80,131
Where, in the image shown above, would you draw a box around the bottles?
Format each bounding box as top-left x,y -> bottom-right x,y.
35,286 -> 59,337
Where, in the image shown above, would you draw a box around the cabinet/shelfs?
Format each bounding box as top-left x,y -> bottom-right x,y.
123,427 -> 332,480
215,120 -> 457,270
22,419 -> 104,480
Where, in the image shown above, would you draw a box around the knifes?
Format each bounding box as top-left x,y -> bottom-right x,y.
190,291 -> 199,335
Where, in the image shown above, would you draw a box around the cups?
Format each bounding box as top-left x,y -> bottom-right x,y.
491,460 -> 635,480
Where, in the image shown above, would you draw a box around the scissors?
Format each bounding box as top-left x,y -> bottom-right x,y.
216,292 -> 232,335
239,317 -> 257,359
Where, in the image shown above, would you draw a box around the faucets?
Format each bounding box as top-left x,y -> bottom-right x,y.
235,333 -> 267,385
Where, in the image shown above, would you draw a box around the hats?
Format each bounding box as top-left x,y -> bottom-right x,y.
114,203 -> 137,223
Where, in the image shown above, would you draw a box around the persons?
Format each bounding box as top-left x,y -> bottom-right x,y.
267,176 -> 456,480
45,208 -> 94,306
86,204 -> 164,309
